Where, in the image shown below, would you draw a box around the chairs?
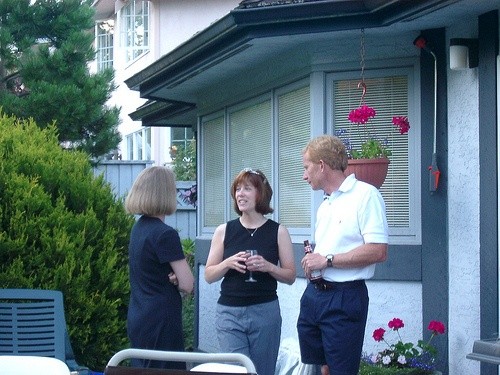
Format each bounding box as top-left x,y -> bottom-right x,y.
0,288 -> 65,362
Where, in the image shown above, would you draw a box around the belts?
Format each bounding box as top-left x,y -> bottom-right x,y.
315,279 -> 364,290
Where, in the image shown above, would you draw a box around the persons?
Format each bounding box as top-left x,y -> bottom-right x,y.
204,167 -> 298,375
296,134 -> 389,375
125,165 -> 195,370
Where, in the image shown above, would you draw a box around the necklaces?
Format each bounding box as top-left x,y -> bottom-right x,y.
242,227 -> 260,237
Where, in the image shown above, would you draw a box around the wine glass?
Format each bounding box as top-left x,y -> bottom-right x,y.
244,249 -> 258,283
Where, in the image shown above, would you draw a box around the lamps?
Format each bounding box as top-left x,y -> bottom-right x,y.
449,38 -> 480,70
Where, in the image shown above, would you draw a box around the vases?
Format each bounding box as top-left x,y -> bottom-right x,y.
369,174 -> 372,175
343,159 -> 391,189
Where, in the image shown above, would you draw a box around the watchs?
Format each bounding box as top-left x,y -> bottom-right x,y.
325,253 -> 334,267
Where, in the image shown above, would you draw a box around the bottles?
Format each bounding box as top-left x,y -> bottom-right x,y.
304,239 -> 322,283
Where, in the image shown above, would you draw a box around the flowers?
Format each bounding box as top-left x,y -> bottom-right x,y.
334,106 -> 410,159
361,318 -> 445,375
